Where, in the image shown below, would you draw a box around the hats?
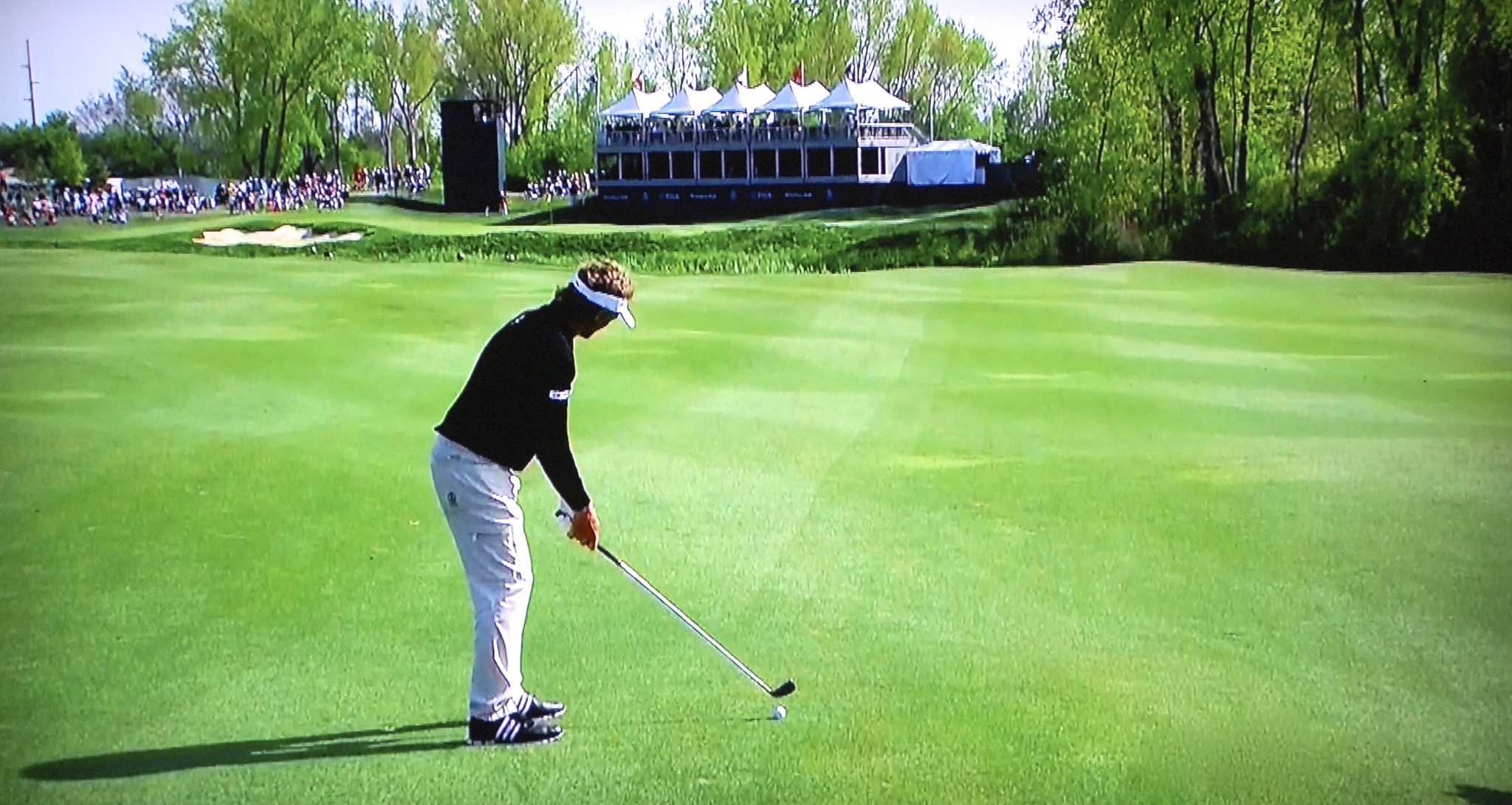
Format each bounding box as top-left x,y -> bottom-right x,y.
572,271 -> 638,330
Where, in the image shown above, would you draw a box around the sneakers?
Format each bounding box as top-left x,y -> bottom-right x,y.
507,694 -> 564,722
467,710 -> 564,749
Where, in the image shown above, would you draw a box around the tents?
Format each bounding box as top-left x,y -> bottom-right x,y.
811,77 -> 910,140
905,139 -> 1001,183
599,90 -> 672,144
657,87 -> 723,143
700,82 -> 777,142
751,81 -> 833,142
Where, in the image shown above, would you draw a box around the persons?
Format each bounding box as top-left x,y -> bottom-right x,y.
1,159 -> 601,229
594,108 -> 905,146
429,256 -> 636,748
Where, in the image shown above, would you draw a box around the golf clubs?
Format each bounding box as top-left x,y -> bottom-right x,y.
557,511 -> 797,698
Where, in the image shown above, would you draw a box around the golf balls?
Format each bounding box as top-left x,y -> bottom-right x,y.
774,705 -> 787,719
